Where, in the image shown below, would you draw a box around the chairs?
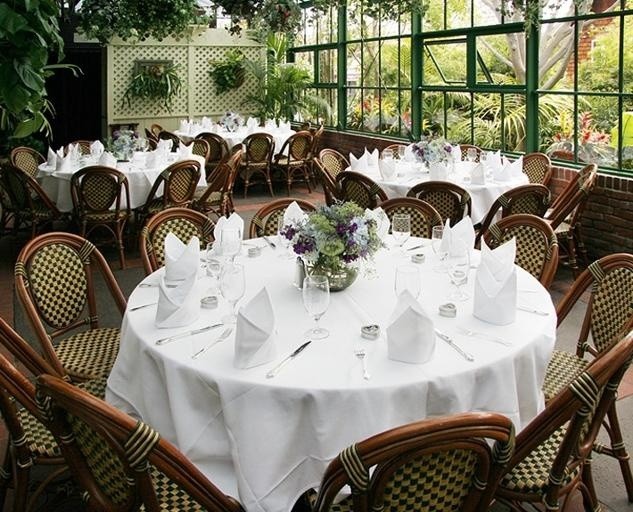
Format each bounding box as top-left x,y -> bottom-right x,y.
2,167 -> 55,268
373,199 -> 442,237
472,209 -> 557,286
143,204 -> 215,277
72,170 -> 135,268
532,253 -> 633,504
253,197 -> 320,239
545,164 -> 600,281
9,123 -> 554,226
294,412 -> 515,509
489,326 -> 633,508
16,234 -> 127,376
475,184 -> 550,247
0,320 -> 88,509
39,373 -> 246,512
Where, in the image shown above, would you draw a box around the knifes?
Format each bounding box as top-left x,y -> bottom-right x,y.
267,339 -> 313,379
156,323 -> 226,345
434,326 -> 476,363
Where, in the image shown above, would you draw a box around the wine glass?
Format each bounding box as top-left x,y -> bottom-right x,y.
301,274 -> 331,340
392,215 -> 472,301
207,226 -> 247,324
398,144 -> 407,163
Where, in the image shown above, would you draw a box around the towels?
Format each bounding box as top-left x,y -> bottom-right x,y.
180,117 -> 291,133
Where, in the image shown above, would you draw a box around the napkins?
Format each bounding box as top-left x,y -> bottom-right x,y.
213,211 -> 246,256
471,236 -> 520,326
364,206 -> 390,246
282,200 -> 308,246
386,290 -> 438,364
156,275 -> 201,329
164,232 -> 203,278
234,285 -> 278,367
439,216 -> 475,258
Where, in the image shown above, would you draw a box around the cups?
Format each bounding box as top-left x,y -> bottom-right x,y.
467,148 -> 477,163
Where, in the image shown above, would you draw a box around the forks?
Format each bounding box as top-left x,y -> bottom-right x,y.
352,343 -> 373,383
190,327 -> 233,362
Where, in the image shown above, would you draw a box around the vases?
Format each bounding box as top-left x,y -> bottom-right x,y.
303,258 -> 360,294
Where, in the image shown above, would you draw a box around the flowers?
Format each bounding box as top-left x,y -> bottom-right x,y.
221,111 -> 245,126
279,199 -> 387,261
